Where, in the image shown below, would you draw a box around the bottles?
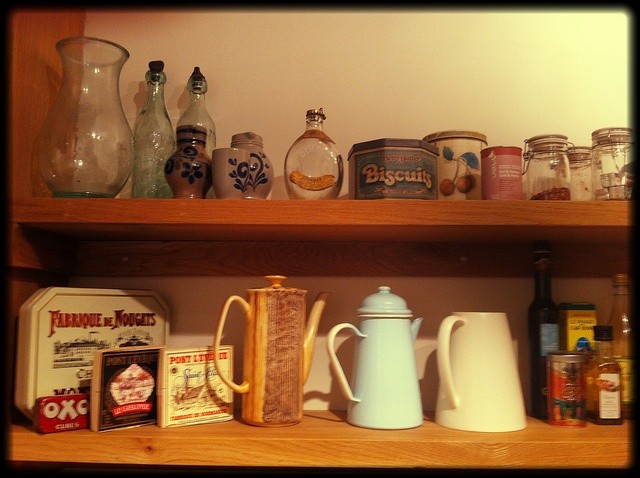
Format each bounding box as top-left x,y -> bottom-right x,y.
177,66 -> 217,163
527,240 -> 560,420
609,272 -> 633,420
283,108 -> 344,200
132,60 -> 176,198
231,132 -> 274,199
164,124 -> 213,200
586,324 -> 624,426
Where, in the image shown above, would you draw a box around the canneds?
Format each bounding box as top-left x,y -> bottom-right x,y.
481,147 -> 524,202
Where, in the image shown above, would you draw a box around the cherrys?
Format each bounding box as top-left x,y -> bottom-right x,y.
439,146 -> 477,194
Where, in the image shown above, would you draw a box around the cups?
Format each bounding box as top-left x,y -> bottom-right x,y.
212,148 -> 250,199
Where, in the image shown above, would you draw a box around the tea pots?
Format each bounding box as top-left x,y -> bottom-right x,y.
213,273 -> 330,428
325,285 -> 424,429
434,310 -> 529,432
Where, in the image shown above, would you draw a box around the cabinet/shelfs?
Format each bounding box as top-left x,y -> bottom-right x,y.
4,193 -> 635,471
